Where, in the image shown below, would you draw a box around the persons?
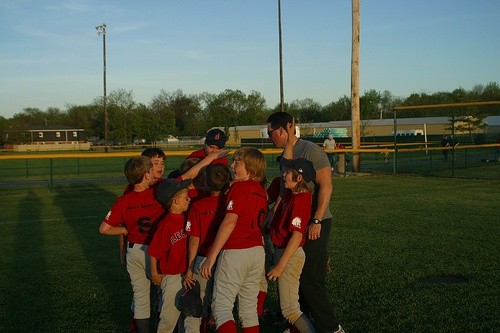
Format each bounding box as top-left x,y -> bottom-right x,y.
146,178 -> 192,333
264,111 -> 346,333
119,148 -> 178,333
267,116 -> 317,333
98,149 -> 232,333
440,134 -> 460,162
182,163 -> 231,333
199,147 -> 270,333
323,133 -> 336,171
167,128 -> 331,317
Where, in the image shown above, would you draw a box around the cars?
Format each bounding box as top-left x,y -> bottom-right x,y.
165,135 -> 178,141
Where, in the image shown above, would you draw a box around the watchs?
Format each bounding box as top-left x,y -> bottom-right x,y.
312,218 -> 322,224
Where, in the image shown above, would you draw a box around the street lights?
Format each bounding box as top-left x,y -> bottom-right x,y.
94,23 -> 109,152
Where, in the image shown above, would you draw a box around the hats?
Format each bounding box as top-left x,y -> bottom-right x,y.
173,279 -> 205,320
206,128 -> 227,149
275,155 -> 317,184
155,178 -> 193,204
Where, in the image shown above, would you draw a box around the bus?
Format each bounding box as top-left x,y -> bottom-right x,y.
392,129 -> 426,138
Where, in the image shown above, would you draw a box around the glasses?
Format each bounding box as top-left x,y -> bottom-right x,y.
267,126 -> 281,137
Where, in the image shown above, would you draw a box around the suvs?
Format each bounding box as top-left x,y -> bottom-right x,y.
134,137 -> 146,144
